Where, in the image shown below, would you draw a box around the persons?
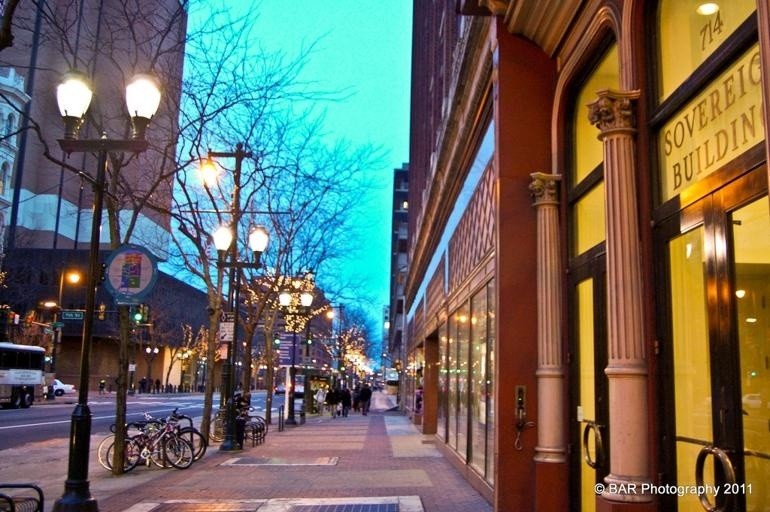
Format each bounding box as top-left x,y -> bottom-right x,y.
98,376 -> 160,395
313,382 -> 372,419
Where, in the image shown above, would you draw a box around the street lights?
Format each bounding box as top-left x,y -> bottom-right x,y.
46,263 -> 82,400
145,345 -> 159,393
210,216 -> 266,450
201,143 -> 251,444
281,278 -> 342,422
49,68 -> 165,510
178,352 -> 189,386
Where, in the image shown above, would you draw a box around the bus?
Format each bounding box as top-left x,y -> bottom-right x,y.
277,365 -> 313,396
0,342 -> 46,410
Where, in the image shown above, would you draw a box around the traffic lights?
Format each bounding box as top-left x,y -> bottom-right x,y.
275,334 -> 280,348
134,302 -> 144,324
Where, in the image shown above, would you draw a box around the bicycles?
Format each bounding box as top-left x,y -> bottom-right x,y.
97,407 -> 207,471
208,397 -> 267,442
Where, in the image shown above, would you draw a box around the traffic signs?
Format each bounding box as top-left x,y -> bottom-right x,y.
63,312 -> 83,320
50,322 -> 64,327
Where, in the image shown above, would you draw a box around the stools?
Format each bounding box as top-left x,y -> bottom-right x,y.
0,483 -> 45,512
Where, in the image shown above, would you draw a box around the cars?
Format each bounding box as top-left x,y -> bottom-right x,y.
43,379 -> 76,397
